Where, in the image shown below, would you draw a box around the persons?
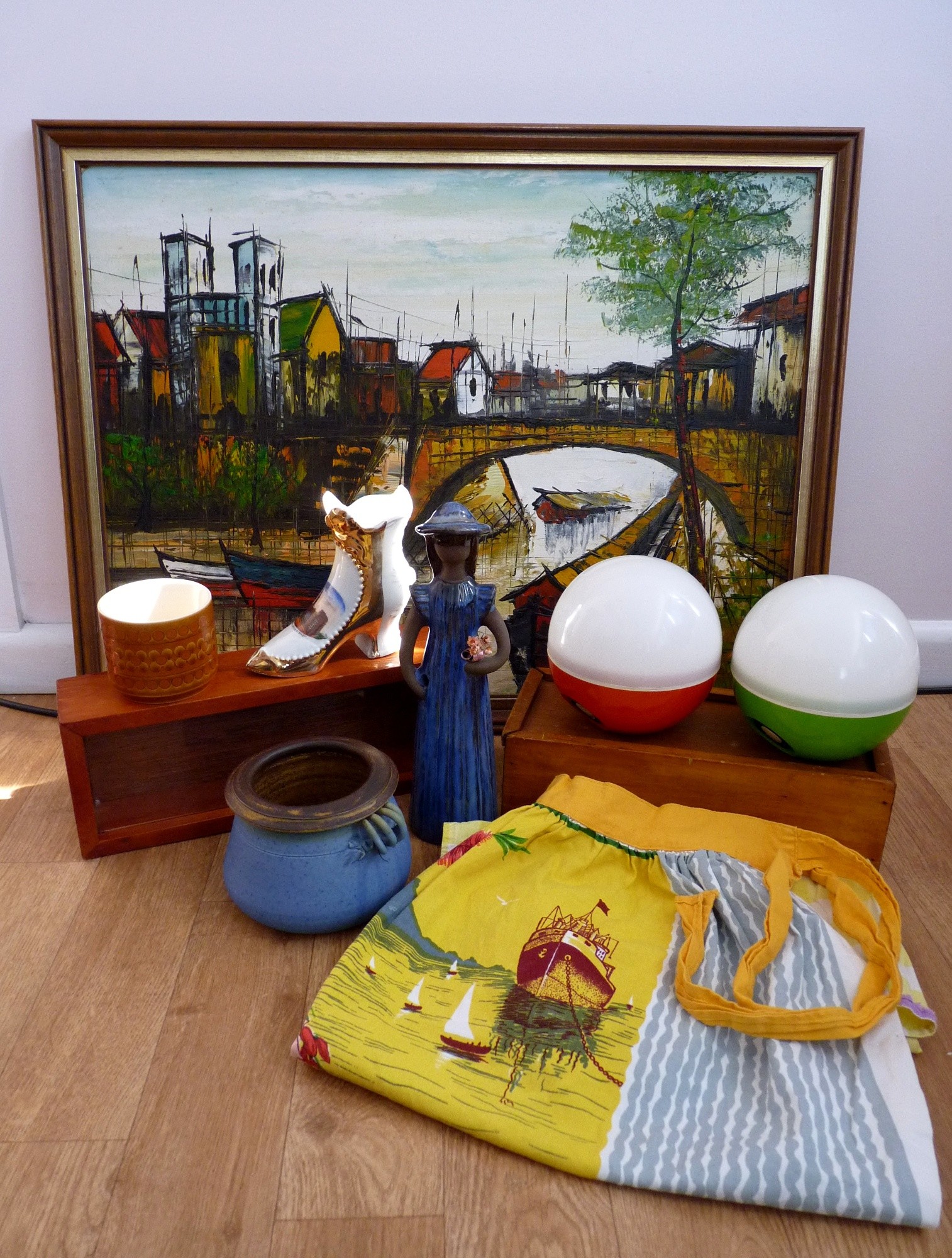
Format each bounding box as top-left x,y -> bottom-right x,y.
399,502 -> 511,845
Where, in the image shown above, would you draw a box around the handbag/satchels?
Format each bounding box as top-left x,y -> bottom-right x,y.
289,774 -> 943,1231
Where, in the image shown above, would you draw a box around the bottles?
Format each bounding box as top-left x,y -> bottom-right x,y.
222,737 -> 411,934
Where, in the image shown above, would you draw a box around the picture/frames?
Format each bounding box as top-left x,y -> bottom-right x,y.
29,116 -> 865,729
